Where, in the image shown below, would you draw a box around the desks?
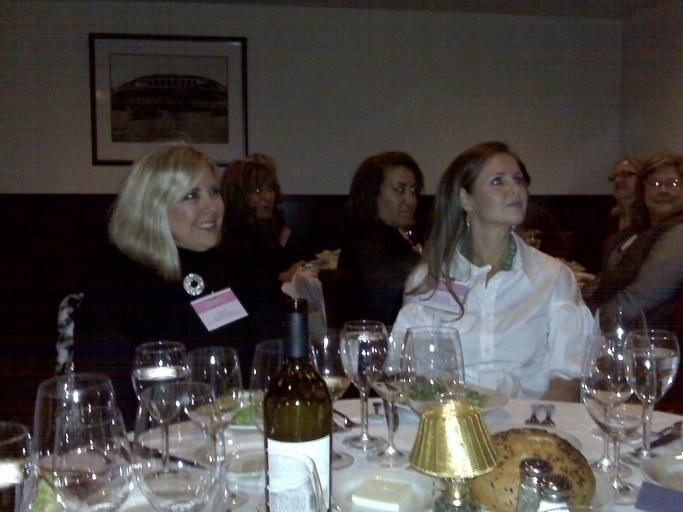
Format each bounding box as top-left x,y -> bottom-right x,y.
33,397 -> 683,512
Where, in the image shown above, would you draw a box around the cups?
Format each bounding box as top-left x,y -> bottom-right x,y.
0,422 -> 40,510
33,372 -> 132,511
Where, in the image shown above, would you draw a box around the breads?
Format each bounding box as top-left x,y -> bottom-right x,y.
470,428 -> 596,512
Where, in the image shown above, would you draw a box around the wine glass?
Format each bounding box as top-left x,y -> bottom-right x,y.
249,319 -> 464,467
129,339 -> 249,511
573,300 -> 681,504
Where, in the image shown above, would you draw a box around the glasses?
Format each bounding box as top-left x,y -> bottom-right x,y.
641,177 -> 683,190
608,169 -> 639,182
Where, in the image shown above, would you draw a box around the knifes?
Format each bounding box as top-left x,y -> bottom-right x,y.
127,439 -> 206,470
634,433 -> 682,455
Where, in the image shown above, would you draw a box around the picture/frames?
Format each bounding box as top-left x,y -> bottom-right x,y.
89,33 -> 248,166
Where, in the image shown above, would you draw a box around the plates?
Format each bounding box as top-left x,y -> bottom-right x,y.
184,382 -> 508,431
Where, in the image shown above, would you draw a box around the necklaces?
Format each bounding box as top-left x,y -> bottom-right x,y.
456,225 -> 523,275
170,250 -> 210,296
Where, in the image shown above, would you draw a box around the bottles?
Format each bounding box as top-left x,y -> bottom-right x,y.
513,457 -> 573,512
262,296 -> 331,508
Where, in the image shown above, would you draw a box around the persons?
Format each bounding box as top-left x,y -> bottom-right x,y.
597,149 -> 681,415
384,139 -> 600,402
72,141 -> 287,443
516,205 -> 556,254
337,150 -> 424,339
222,153 -> 341,384
581,155 -> 642,291
